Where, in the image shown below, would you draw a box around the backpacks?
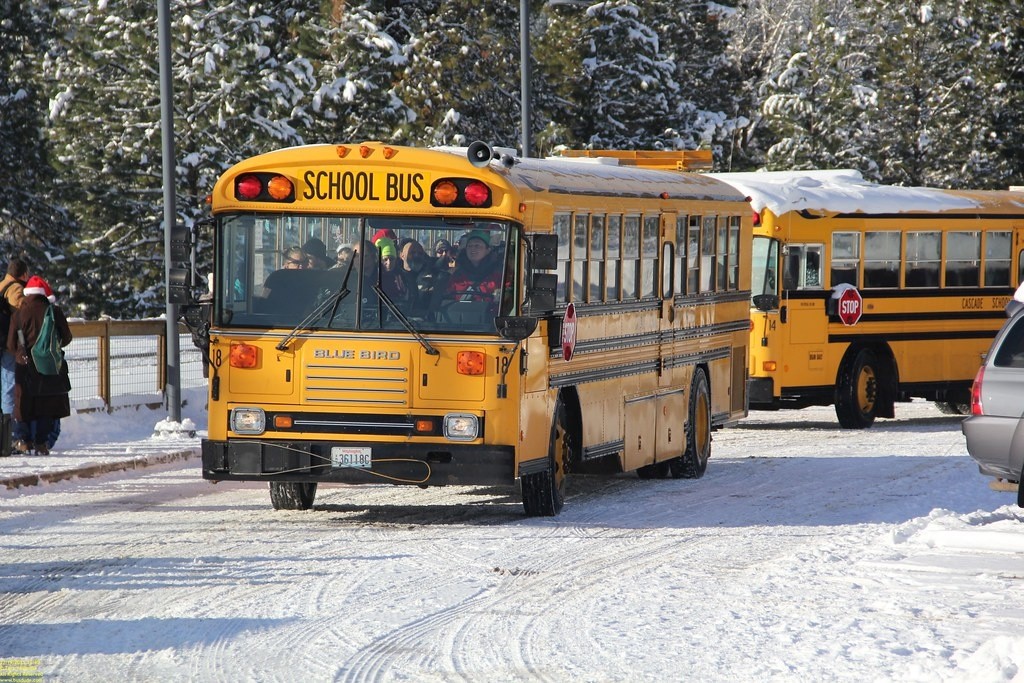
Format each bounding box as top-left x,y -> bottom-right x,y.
0,281 -> 25,350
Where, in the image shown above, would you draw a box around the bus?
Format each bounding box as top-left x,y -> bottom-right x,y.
169,141 -> 753,518
697,170 -> 1024,429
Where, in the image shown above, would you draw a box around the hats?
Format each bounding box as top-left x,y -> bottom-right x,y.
302,238 -> 326,257
336,243 -> 352,252
375,237 -> 397,259
23,276 -> 56,303
467,231 -> 490,245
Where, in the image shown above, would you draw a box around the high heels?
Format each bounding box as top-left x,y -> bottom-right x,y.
16,442 -> 31,455
34,440 -> 49,456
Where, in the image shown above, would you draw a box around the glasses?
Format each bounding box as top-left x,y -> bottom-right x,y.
435,247 -> 452,254
287,260 -> 309,266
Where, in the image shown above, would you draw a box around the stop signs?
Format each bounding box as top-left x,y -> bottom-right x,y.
839,288 -> 862,326
562,303 -> 577,362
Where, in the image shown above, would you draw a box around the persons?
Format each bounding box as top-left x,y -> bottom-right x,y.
6,275 -> 72,456
250,227 -> 515,324
0,257 -> 30,457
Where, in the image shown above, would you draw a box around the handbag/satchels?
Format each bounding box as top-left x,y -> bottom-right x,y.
31,304 -> 63,376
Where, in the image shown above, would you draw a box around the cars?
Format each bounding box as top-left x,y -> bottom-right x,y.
959,283 -> 1023,510
